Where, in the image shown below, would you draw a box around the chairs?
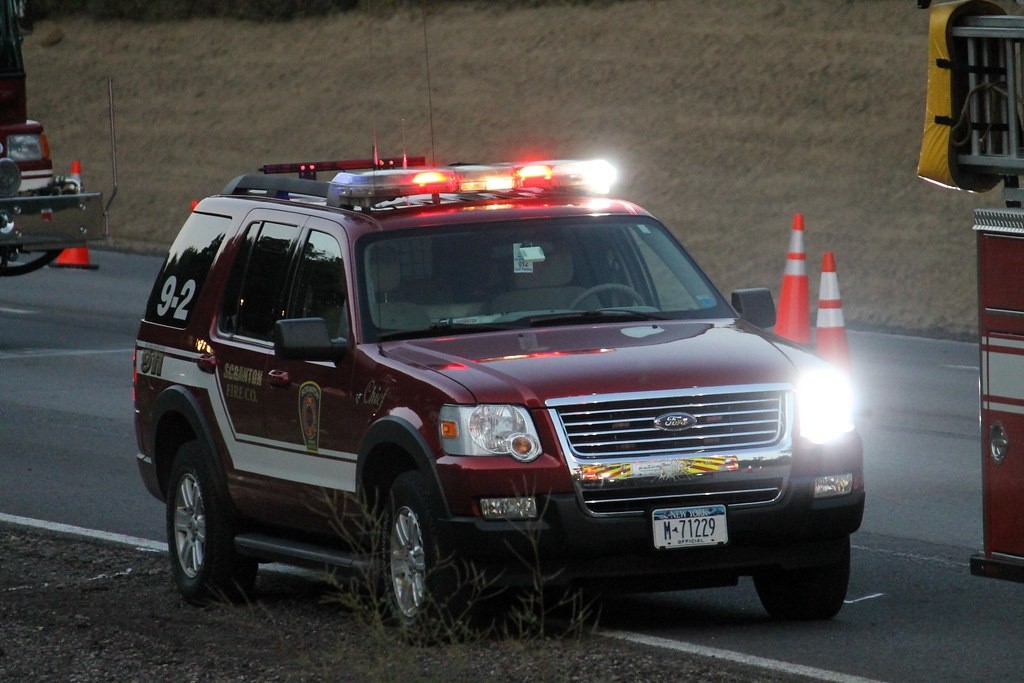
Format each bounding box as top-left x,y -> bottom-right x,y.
492,248 -> 603,316
322,246 -> 429,338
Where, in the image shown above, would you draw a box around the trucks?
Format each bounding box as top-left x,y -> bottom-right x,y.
0,0 -> 106,277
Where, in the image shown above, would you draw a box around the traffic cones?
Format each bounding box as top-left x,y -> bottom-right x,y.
52,160 -> 100,271
773,213 -> 812,344
813,252 -> 853,367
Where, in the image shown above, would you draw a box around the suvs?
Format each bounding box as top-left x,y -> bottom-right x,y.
129,153 -> 867,647
